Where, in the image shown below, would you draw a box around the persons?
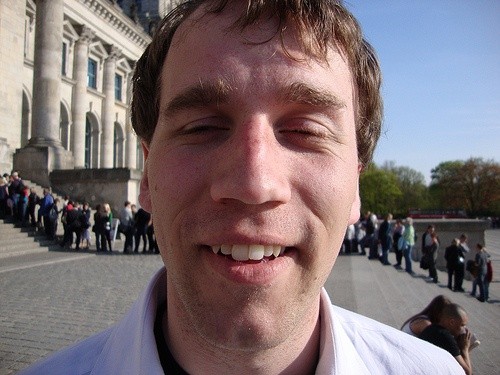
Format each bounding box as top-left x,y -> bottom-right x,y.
475,243 -> 488,301
402,218 -> 414,273
470,247 -> 492,299
400,295 -> 453,338
62,194 -> 92,251
121,201 -> 160,254
340,210 -> 405,267
421,224 -> 439,278
16,0 -> 467,375
0,171 -> 53,236
46,198 -> 63,241
459,234 -> 469,287
419,303 -> 472,375
444,238 -> 465,293
93,203 -> 112,254
421,234 -> 440,284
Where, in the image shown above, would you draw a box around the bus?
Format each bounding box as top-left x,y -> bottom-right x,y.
406,207 -> 467,219
